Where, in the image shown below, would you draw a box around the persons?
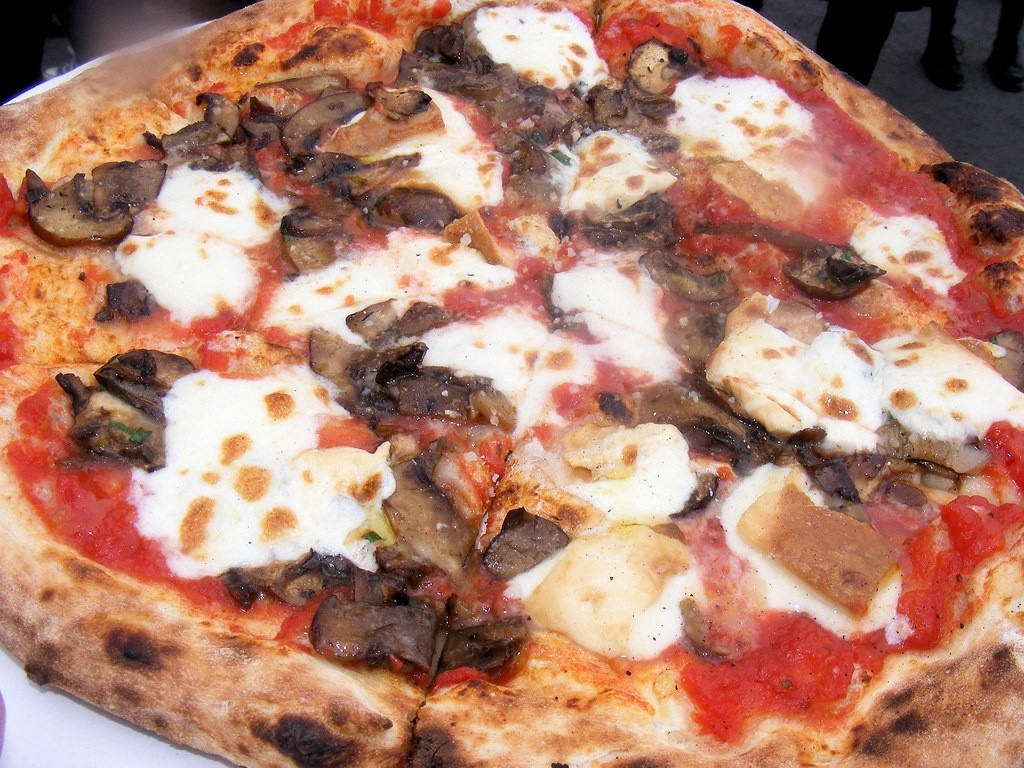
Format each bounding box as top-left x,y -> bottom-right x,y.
734,0 -> 898,89
919,0 -> 1024,91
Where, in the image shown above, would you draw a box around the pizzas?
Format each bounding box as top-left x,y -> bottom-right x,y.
0,0 -> 1024,768
